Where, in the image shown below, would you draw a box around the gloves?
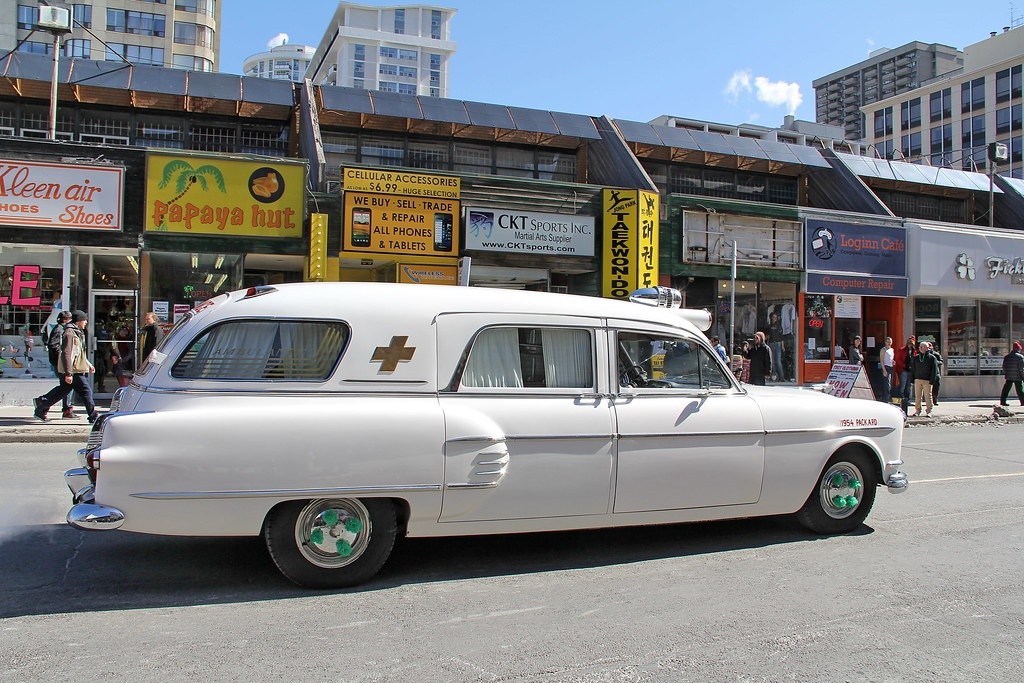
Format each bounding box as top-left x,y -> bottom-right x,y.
633,372 -> 648,385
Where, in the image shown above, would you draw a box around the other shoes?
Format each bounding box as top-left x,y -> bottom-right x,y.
62,408 -> 80,420
32,396 -> 51,423
1000,403 -> 1009,406
926,413 -> 931,418
934,403 -> 939,406
913,411 -> 922,417
87,410 -> 102,423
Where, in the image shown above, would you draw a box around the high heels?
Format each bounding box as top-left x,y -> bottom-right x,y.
0,334 -> 56,381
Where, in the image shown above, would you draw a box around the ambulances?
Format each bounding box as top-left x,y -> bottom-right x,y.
68,279 -> 907,592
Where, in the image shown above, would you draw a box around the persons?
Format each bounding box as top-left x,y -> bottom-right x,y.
707,335 -> 726,368
1000,342 -> 1024,406
743,332 -> 773,386
94,318 -> 134,392
849,336 -> 864,365
733,341 -> 750,355
911,342 -> 943,418
33,311 -> 81,420
139,312 -> 164,366
663,340 -> 691,376
764,312 -> 785,382
880,337 -> 896,405
990,347 -> 1003,375
33,310 -> 99,422
896,335 -> 917,406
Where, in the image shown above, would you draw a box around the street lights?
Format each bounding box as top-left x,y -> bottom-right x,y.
729,240 -> 737,372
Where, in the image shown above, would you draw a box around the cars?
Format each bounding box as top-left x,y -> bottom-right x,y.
41,289 -> 136,343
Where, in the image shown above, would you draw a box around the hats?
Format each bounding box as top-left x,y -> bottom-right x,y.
1013,342 -> 1022,351
68,309 -> 88,323
